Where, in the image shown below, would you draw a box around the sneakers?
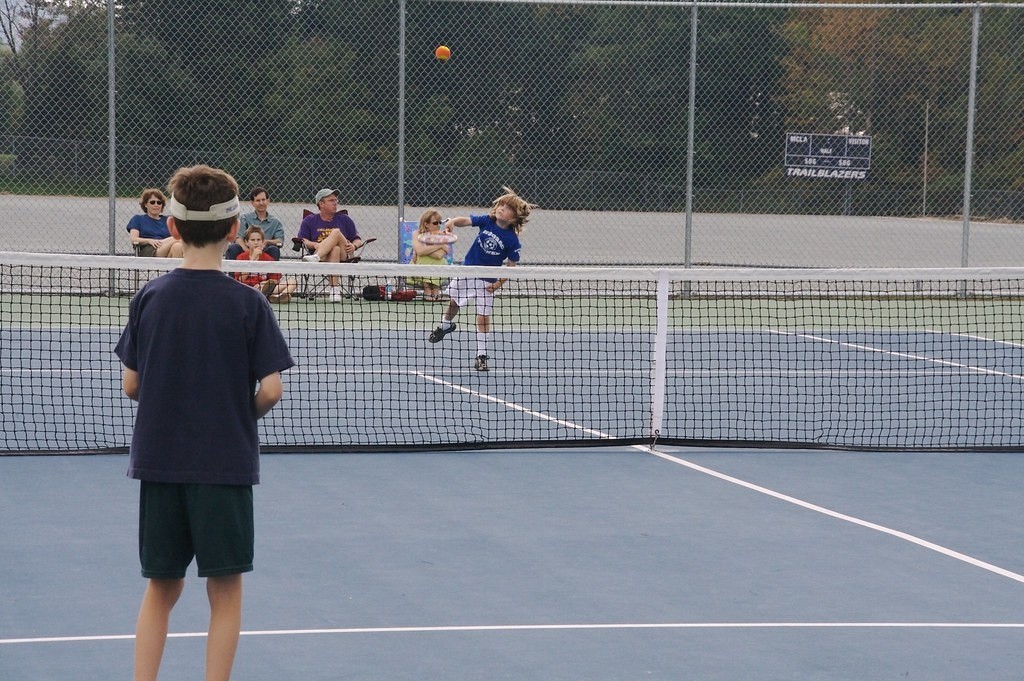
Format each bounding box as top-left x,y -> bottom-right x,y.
302,253 -> 319,261
328,287 -> 342,302
474,354 -> 491,371
428,321 -> 456,343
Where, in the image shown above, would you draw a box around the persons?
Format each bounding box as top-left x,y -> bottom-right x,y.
297,188 -> 364,303
125,188 -> 185,259
233,224 -> 296,303
427,185 -> 539,371
113,162 -> 293,681
224,187 -> 285,282
404,211 -> 450,303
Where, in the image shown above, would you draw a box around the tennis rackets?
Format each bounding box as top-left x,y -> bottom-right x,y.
417,226 -> 458,245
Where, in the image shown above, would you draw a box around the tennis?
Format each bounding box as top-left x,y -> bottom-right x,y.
436,46 -> 449,60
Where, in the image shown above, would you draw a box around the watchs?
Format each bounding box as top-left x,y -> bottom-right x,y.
354,245 -> 358,251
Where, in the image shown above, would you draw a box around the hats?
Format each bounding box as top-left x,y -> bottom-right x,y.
315,188 -> 340,204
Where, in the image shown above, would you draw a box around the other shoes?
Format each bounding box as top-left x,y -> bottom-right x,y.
269,292 -> 291,303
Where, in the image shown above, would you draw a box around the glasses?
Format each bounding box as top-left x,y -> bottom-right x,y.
147,199 -> 162,204
428,220 -> 442,225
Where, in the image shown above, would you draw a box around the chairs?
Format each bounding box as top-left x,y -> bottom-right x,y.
398,217 -> 455,302
291,209 -> 377,302
